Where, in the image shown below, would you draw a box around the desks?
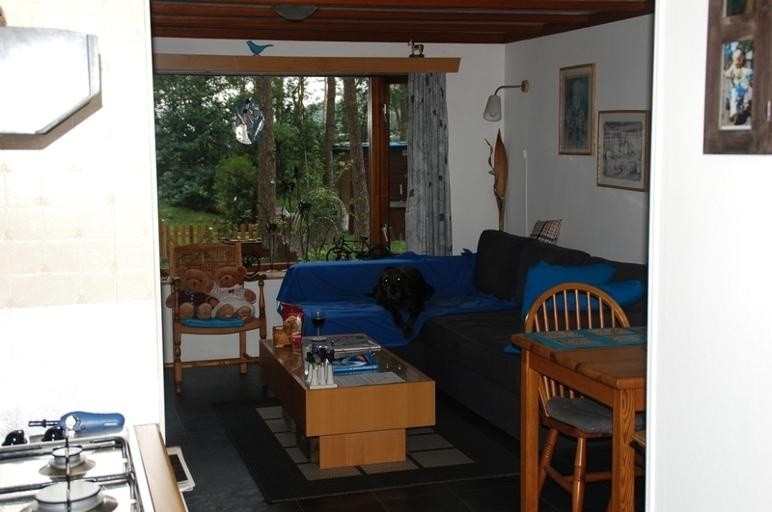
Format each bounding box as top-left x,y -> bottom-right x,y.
511,326 -> 645,512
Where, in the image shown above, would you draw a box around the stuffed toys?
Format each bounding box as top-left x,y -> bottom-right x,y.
166,266 -> 219,320
207,265 -> 256,320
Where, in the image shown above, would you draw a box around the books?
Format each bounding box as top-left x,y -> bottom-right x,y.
327,334 -> 382,352
333,353 -> 378,371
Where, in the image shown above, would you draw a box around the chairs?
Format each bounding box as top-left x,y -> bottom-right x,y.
524,283 -> 645,512
168,240 -> 267,394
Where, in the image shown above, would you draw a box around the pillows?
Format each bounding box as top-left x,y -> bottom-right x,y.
538,279 -> 645,311
530,218 -> 562,244
520,261 -> 616,318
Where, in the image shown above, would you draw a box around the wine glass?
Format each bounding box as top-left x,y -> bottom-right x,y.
311,310 -> 326,341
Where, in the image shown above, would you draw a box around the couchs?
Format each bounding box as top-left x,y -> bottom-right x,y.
276,230 -> 648,452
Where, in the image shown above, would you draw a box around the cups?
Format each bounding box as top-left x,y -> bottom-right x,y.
32,481 -> 103,512
52,447 -> 83,464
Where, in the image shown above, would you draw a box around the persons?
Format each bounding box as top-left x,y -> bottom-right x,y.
722,49 -> 754,124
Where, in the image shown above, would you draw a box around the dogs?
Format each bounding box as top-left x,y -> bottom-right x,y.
408,39 -> 424,56
373,265 -> 434,338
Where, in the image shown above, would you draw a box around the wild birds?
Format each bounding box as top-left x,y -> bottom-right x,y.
246,41 -> 273,56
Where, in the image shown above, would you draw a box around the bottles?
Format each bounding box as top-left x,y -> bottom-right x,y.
28,412 -> 125,430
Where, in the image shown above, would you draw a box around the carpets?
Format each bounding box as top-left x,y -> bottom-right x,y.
210,397 -> 519,505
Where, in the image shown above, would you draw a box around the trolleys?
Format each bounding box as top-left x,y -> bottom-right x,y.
242,240 -> 272,277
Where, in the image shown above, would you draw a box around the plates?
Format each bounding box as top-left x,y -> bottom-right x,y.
48,458 -> 84,469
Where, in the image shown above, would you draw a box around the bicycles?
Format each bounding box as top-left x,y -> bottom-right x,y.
325,236 -> 393,261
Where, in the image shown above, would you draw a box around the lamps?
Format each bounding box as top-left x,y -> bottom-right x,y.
484,80 -> 529,122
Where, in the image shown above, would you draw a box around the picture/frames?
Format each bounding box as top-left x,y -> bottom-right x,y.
558,63 -> 597,156
596,109 -> 649,191
